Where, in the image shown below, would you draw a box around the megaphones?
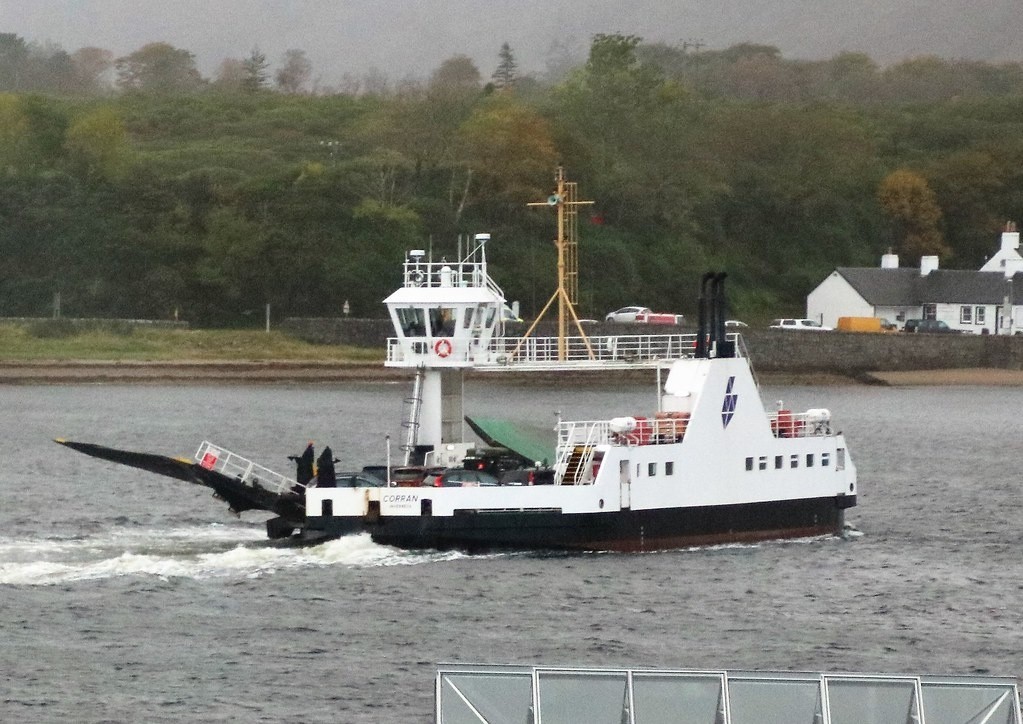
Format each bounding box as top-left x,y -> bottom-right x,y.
547,195 -> 558,206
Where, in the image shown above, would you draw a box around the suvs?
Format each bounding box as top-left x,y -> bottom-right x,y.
769,318 -> 836,331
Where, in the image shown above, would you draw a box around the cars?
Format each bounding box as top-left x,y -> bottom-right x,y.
569,320 -> 601,327
605,306 -> 651,323
724,320 -> 747,328
306,448 -> 556,489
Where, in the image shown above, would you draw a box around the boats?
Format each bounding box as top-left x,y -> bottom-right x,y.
50,165 -> 858,556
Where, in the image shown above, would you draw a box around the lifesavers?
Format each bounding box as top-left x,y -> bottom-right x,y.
435,339 -> 452,357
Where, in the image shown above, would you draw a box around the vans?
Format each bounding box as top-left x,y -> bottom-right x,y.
487,304 -> 524,323
905,318 -> 952,334
